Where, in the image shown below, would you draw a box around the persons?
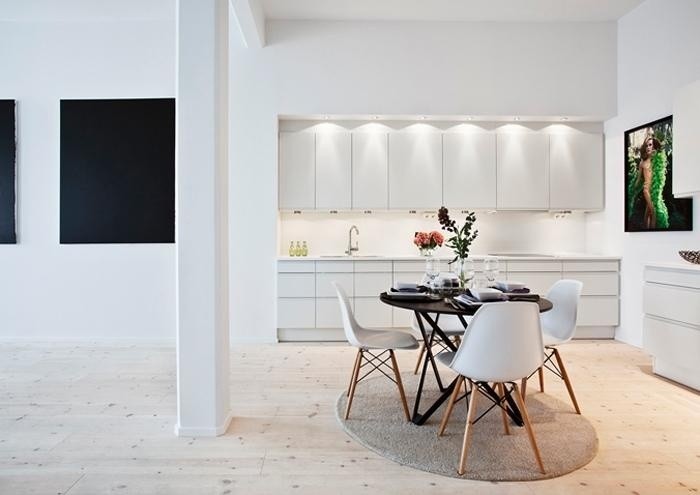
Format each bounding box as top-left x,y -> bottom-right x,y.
633,135 -> 661,229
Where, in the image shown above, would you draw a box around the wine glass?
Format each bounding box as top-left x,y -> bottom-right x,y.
425,258 -> 439,299
458,255 -> 499,288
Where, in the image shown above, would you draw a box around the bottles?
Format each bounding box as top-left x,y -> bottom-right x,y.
288,241 -> 308,257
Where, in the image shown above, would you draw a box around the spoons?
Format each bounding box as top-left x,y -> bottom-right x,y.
445,297 -> 458,309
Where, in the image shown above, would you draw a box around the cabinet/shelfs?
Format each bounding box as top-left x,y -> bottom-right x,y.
495,136 -> 605,209
507,261 -> 621,340
279,260 -> 392,343
389,134 -> 498,208
640,261 -> 700,393
279,129 -> 389,212
670,82 -> 699,198
393,261 -> 506,329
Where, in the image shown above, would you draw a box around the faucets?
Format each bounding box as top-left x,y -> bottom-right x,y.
345,224 -> 360,255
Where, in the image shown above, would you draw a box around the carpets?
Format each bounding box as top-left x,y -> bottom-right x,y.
337,369 -> 601,482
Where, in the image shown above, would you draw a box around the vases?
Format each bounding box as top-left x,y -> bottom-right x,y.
457,257 -> 470,292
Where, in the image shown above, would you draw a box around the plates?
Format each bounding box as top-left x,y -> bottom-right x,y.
454,294 -> 510,306
387,290 -> 427,301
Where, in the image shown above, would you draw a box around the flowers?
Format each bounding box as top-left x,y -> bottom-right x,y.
414,231 -> 446,251
437,204 -> 479,286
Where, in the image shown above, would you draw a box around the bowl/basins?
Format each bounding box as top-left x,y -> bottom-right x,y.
471,287 -> 502,299
496,280 -> 526,293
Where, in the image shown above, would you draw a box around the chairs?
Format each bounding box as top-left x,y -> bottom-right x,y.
522,277 -> 583,415
410,270 -> 471,375
433,299 -> 545,477
334,287 -> 421,421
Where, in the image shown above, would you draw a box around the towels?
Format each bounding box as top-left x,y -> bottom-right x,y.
386,281 -> 541,312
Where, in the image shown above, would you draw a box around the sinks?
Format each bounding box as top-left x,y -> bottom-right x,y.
319,255 -> 381,258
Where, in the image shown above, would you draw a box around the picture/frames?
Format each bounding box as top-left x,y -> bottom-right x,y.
623,114 -> 695,233
